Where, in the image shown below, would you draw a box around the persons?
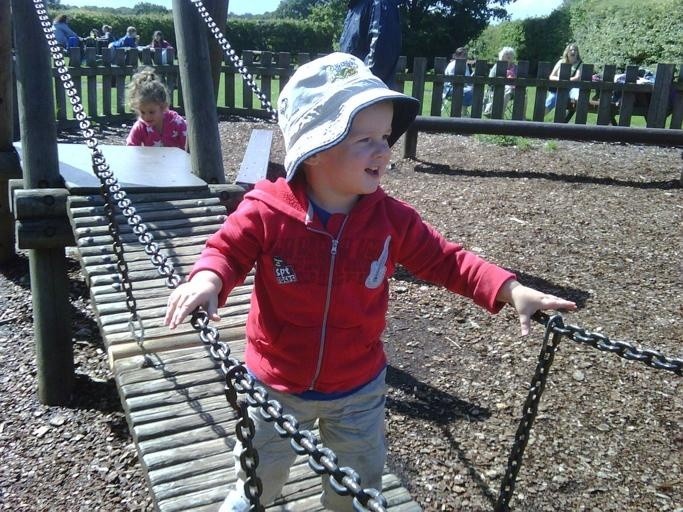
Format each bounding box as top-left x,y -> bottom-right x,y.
124,68 -> 190,154
543,43 -> 582,115
442,47 -> 472,107
483,46 -> 531,121
162,52 -> 575,511
339,1 -> 404,90
51,15 -> 173,66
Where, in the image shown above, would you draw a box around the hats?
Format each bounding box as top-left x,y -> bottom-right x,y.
276,51 -> 420,183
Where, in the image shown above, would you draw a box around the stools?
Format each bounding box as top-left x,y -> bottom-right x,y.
440,94 -> 470,117
487,88 -> 515,119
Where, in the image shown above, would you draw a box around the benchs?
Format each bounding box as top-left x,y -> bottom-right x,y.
564,98 -> 671,126
